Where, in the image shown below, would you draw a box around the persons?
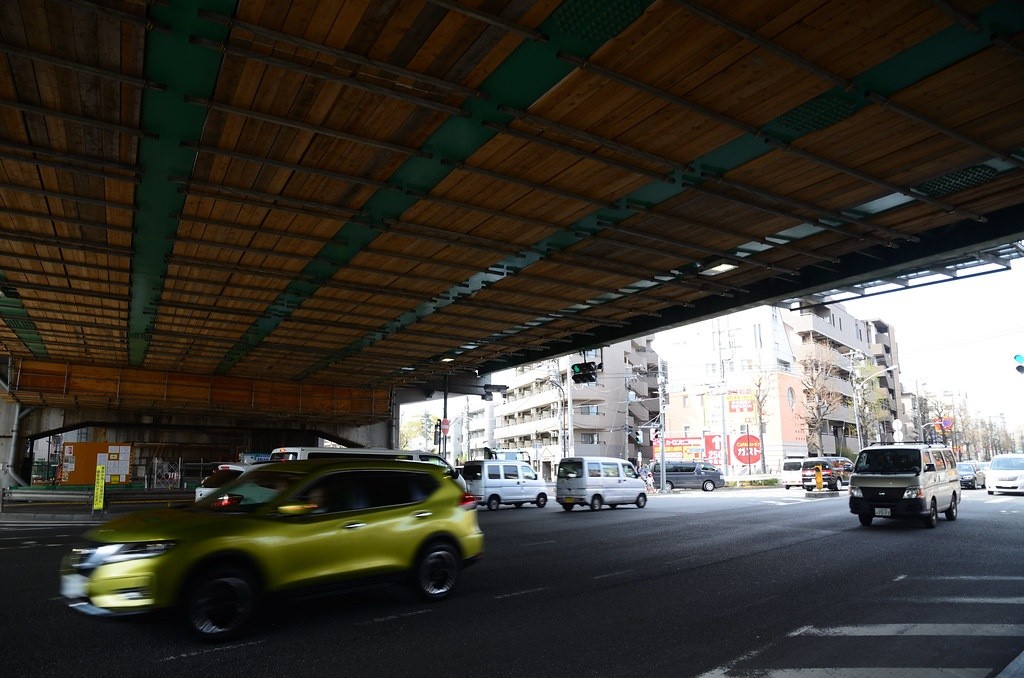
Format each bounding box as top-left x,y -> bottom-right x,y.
864,454 -> 887,470
310,491 -> 329,513
902,455 -> 919,470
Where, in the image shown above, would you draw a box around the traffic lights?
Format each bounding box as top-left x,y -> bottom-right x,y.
1014,354 -> 1024,374
571,361 -> 597,384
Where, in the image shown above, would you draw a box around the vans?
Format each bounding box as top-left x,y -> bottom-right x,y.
557,454 -> 647,512
649,460 -> 725,491
463,459 -> 550,511
848,439 -> 963,529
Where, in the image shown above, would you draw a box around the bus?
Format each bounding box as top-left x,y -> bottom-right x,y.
270,448 -> 468,497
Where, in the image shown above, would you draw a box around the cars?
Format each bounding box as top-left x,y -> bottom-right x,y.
801,454 -> 855,493
49,458 -> 486,643
956,459 -> 985,489
193,459 -> 250,506
984,453 -> 1024,495
781,457 -> 804,489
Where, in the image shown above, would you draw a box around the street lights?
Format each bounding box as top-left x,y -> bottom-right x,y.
919,421 -> 943,443
854,365 -> 899,448
534,377 -> 567,459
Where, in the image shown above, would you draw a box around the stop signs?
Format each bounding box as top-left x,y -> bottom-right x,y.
441,419 -> 449,435
